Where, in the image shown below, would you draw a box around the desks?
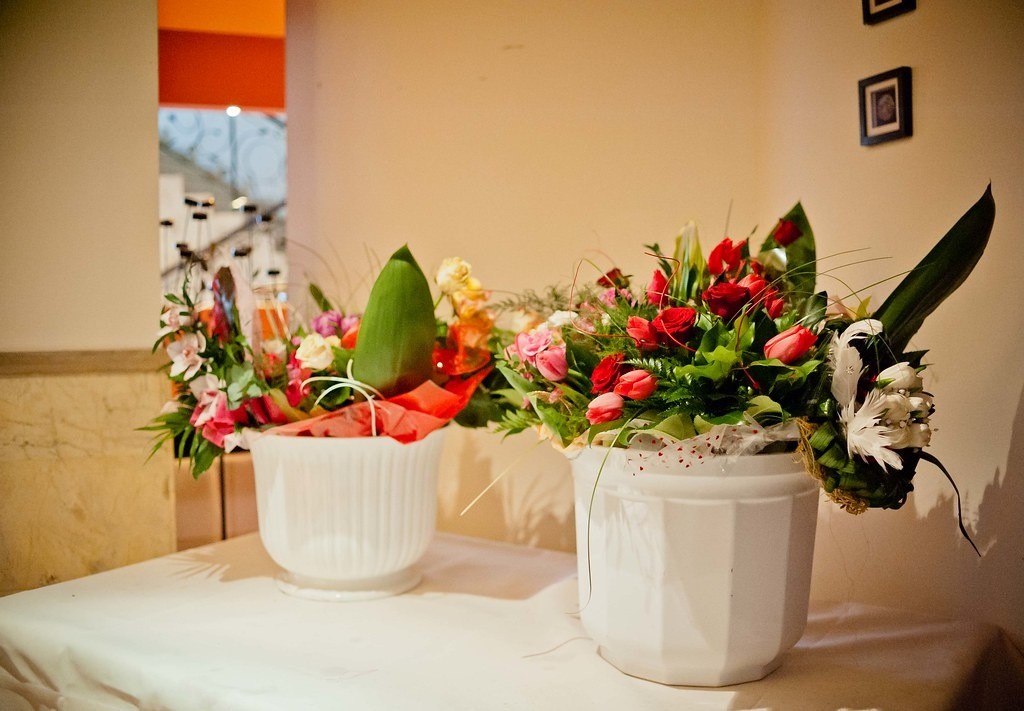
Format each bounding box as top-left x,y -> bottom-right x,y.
1,526 -> 1024,711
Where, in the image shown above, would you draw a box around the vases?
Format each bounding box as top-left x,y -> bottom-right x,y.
236,429 -> 444,593
571,437 -> 820,682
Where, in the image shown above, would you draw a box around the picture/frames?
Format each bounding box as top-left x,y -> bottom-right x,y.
861,0 -> 916,25
858,65 -> 913,145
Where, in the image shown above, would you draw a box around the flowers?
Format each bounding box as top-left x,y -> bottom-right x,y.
484,175 -> 996,557
141,241 -> 504,479
